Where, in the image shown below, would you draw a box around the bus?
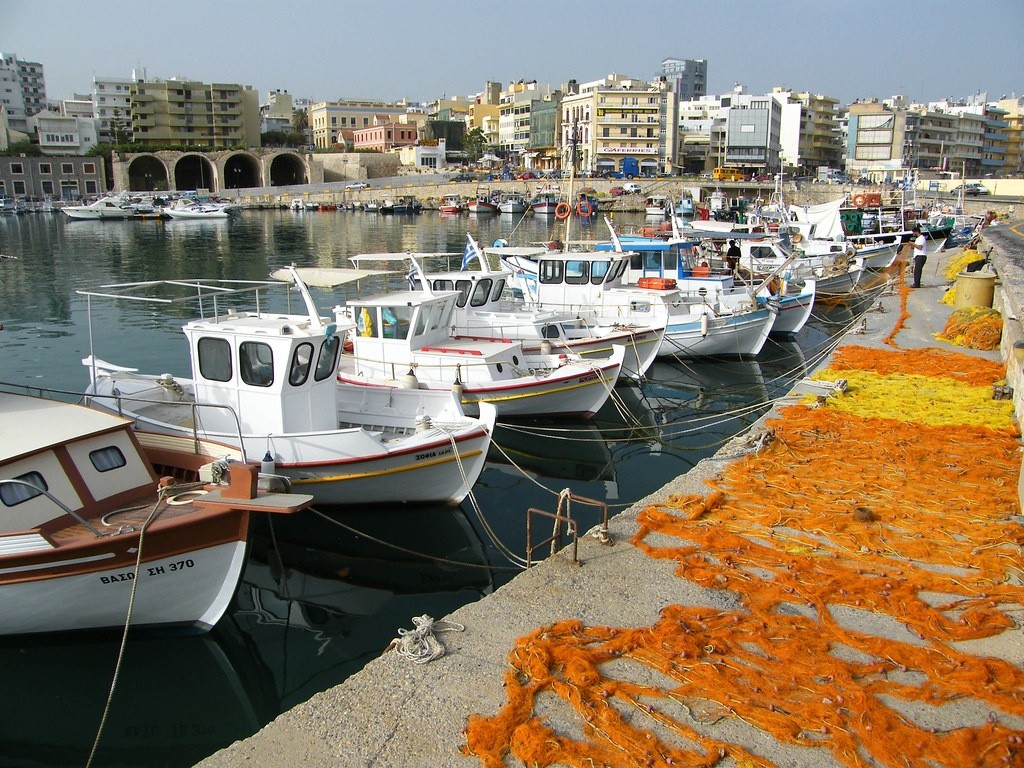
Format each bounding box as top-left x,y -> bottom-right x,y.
713,167 -> 744,182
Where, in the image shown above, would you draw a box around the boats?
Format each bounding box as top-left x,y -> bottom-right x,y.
0,380 -> 313,644
0,106 -> 993,426
75,259 -> 501,514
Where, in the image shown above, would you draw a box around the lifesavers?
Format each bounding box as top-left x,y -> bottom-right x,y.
853,194 -> 866,206
904,209 -> 914,220
577,200 -> 592,217
555,202 -> 571,219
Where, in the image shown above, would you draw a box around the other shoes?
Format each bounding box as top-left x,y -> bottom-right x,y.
910,284 -> 920,288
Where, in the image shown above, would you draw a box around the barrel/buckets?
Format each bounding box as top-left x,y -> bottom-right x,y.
955,272 -> 996,316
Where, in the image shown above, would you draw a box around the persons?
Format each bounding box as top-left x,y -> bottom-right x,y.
908,227 -> 927,288
982,211 -> 994,229
726,240 -> 741,275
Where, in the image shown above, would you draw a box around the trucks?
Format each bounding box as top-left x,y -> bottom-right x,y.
601,156 -> 639,180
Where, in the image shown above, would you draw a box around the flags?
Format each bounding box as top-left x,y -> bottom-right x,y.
408,259 -> 418,287
460,239 -> 478,271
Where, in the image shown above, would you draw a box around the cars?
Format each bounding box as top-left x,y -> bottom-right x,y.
950,184 -> 980,197
560,170 -> 571,178
345,181 -> 370,190
514,172 -> 536,181
449,174 -> 476,182
26,195 -> 39,202
700,174 -> 713,180
638,172 -> 646,178
970,183 -> 989,195
